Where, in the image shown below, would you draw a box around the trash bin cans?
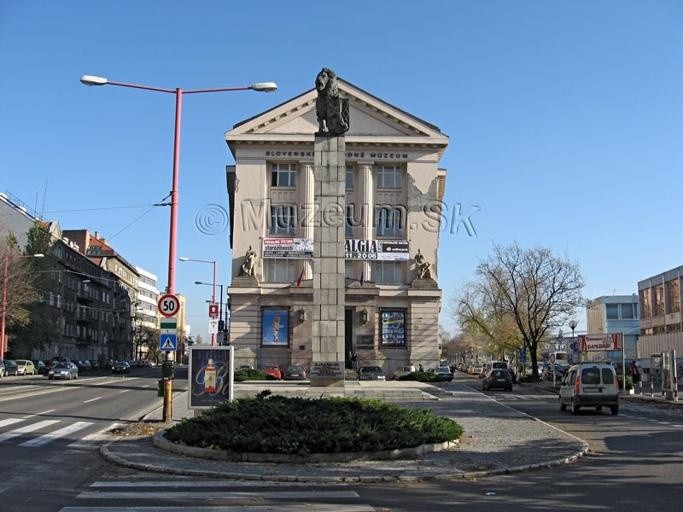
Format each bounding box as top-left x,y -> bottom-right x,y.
157,380 -> 164,397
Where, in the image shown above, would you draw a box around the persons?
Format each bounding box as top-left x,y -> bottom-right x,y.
614,359 -> 641,395
418,363 -> 423,372
271,316 -> 284,343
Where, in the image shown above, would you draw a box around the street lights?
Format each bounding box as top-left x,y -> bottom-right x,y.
450,343 -> 497,364
204,300 -> 226,346
78,75 -> 276,422
194,281 -> 222,346
75,294 -> 156,360
0,253 -> 44,361
58,279 -> 90,361
569,320 -> 576,363
178,257 -> 216,346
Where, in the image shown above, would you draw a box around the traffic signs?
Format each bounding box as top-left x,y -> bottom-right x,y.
158,293 -> 181,317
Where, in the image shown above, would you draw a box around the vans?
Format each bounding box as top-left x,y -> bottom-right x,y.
558,361 -> 619,415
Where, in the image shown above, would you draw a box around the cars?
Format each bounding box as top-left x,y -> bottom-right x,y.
233,363 -> 255,372
355,365 -> 386,380
282,363 -> 308,380
0,356 -> 161,380
481,369 -> 512,392
435,366 -> 453,382
390,365 -> 417,381
440,359 -> 572,381
259,364 -> 283,380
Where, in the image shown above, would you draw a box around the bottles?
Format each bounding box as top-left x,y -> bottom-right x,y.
203,358 -> 216,393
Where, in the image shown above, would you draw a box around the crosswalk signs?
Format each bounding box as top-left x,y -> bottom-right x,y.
160,334 -> 178,351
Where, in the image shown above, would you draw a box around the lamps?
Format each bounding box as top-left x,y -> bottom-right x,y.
297,307 -> 305,323
360,307 -> 368,325
